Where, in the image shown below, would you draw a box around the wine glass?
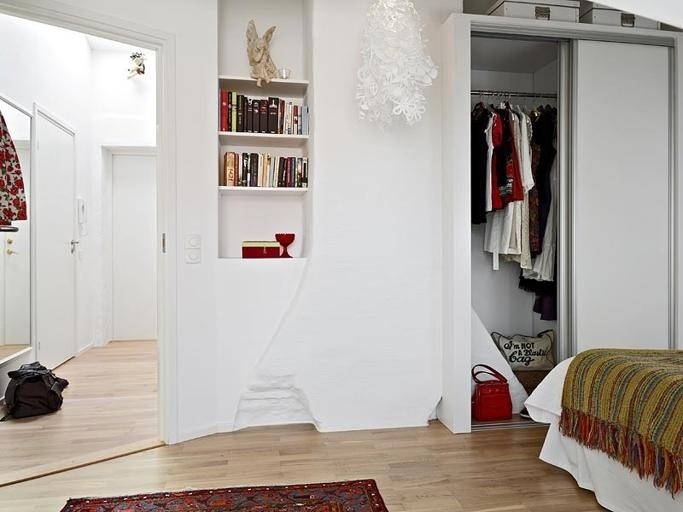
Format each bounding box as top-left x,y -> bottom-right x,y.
275,233 -> 296,258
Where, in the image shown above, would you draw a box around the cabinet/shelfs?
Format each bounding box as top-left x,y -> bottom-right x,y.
218,74 -> 310,258
437,12 -> 683,435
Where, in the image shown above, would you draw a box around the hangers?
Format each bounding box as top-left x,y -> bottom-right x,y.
480,89 -> 547,108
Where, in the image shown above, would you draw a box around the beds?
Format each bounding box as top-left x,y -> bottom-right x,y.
522,348 -> 683,512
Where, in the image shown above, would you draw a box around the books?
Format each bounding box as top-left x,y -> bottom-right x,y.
221,88 -> 309,188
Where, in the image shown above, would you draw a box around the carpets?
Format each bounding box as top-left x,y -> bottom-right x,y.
59,480 -> 387,512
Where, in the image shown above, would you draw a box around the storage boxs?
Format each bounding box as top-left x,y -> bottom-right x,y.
485,0 -> 661,28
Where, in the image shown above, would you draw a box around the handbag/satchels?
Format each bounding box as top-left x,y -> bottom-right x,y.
4,361 -> 69,420
491,330 -> 555,371
471,380 -> 513,423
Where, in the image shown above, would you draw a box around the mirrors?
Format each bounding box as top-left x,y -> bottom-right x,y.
0,93 -> 32,363
